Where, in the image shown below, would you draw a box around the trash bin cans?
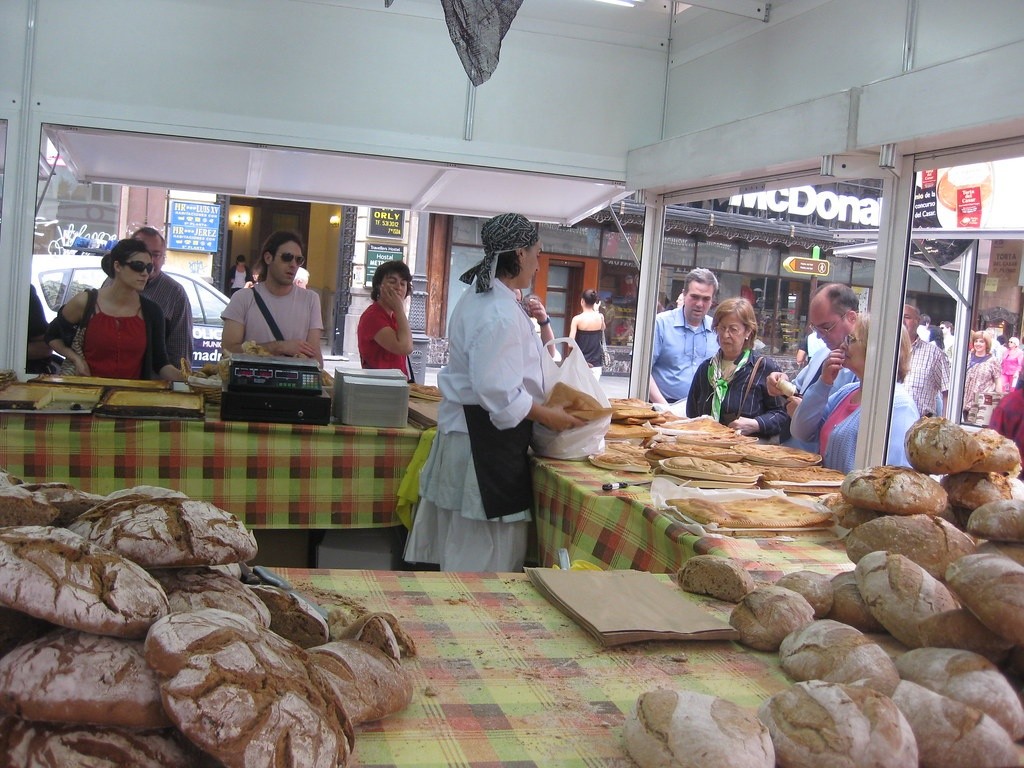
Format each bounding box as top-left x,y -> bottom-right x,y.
405,332 -> 430,385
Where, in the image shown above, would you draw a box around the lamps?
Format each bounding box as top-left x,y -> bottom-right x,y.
330,216 -> 340,228
233,213 -> 250,228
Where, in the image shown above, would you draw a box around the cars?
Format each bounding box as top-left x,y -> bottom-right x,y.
27,252 -> 234,374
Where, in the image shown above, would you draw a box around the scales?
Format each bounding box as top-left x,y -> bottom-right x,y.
227,352 -> 322,395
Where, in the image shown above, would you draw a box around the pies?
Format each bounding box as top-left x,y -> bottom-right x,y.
408,382 -> 442,397
539,381 -> 848,528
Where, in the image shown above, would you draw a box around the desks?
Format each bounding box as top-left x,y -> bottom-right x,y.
0,375 -> 1023,768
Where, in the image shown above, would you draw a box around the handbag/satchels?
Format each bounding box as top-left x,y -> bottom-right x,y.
599,312 -> 610,367
736,356 -> 781,445
529,335 -> 612,462
58,288 -> 98,375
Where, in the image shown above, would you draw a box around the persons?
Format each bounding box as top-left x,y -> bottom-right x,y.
230,256 -> 260,294
766,283 -> 860,453
600,297 -> 633,345
569,290 -> 606,381
789,317 -> 920,475
403,212 -> 586,574
101,228 -> 194,369
648,269 -> 720,404
685,298 -> 788,445
515,289 -> 556,359
918,315 -> 1024,480
796,324 -> 827,369
358,260 -> 415,383
27,285 -> 53,372
220,232 -> 324,368
293,267 -> 309,289
902,305 -> 950,418
44,239 -> 183,381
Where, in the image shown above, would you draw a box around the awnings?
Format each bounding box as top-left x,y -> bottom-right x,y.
829,229 -> 1024,307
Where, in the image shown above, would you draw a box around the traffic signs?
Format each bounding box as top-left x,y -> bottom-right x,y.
782,256 -> 830,276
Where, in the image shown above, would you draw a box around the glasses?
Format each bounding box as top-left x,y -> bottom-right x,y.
844,334 -> 866,346
152,251 -> 163,259
122,261 -> 154,274
714,326 -> 747,336
274,253 -> 305,265
683,332 -> 708,359
809,306 -> 855,336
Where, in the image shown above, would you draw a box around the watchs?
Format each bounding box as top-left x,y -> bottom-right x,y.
537,316 -> 551,325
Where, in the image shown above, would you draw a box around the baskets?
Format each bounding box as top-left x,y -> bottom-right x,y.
181,358 -> 223,404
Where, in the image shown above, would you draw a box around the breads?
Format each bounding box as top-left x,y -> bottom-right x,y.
193,339 -> 335,387
620,417 -> 1023,768
0,373 -> 204,418
0,468 -> 417,768
